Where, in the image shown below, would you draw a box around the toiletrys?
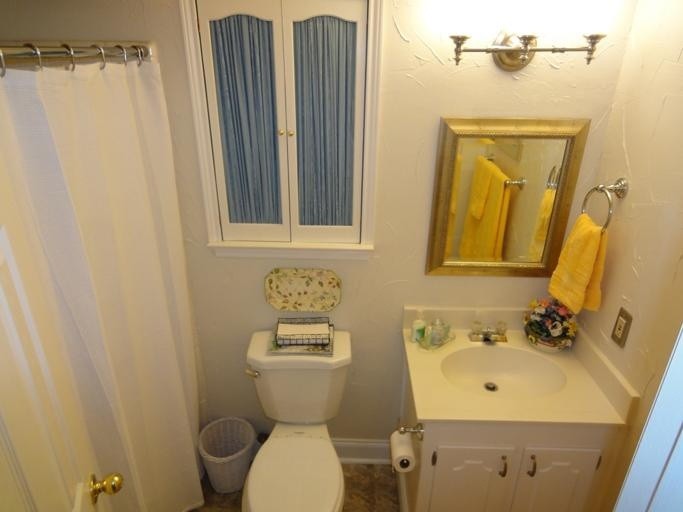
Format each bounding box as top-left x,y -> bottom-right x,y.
410,307 -> 450,347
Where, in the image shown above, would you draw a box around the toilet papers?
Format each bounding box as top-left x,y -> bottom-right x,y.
390,431 -> 416,473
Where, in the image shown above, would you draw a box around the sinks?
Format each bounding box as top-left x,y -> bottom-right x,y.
441,347 -> 567,399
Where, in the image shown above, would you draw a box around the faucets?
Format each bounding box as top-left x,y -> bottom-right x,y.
485,331 -> 508,343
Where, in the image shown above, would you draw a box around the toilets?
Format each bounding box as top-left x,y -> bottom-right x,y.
242,332 -> 353,512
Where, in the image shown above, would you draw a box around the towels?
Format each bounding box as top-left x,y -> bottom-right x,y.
546,214 -> 608,315
529,189 -> 556,262
459,155 -> 512,261
469,158 -> 497,219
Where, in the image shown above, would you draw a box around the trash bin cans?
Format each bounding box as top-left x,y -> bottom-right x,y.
198,416 -> 257,494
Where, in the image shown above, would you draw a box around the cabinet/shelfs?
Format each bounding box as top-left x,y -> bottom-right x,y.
416,423 -> 615,512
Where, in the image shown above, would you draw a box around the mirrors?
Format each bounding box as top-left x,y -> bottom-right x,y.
424,116 -> 592,278
